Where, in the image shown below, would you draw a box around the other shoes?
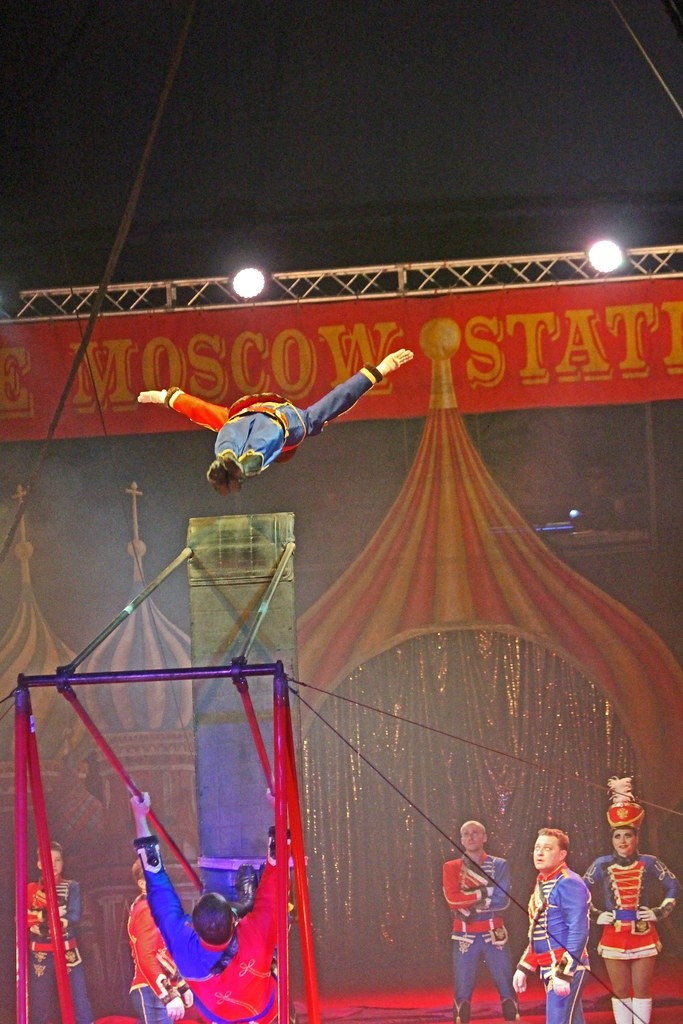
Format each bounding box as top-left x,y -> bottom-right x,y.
206,456 -> 245,496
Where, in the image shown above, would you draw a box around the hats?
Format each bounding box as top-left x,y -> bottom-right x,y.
606,775 -> 646,832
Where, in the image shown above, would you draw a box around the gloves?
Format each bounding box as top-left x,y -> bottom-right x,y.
182,989 -> 194,1008
165,996 -> 185,1022
513,969 -> 528,993
29,924 -> 41,936
137,389 -> 168,404
638,905 -> 658,922
58,904 -> 67,917
596,911 -> 614,925
486,886 -> 495,897
553,977 -> 571,997
376,348 -> 414,376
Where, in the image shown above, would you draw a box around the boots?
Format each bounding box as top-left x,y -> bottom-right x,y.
610,997 -> 652,1024
499,995 -> 520,1021
452,997 -> 472,1024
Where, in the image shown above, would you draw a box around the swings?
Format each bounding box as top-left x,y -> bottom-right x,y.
22,677 -> 280,1024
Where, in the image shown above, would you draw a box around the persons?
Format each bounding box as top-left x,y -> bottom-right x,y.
127,788 -> 297,1024
442,821 -> 520,1024
27,842 -> 95,1024
582,777 -> 683,1024
513,828 -> 591,1024
137,349 -> 414,496
